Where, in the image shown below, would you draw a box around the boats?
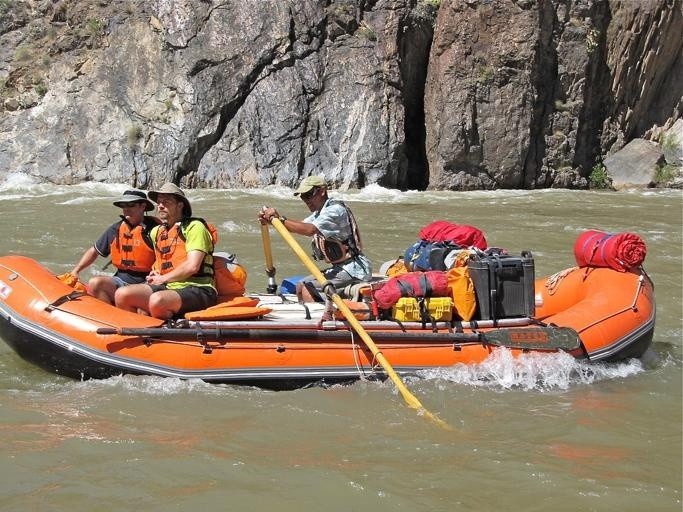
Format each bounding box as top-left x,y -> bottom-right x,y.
0,230 -> 656,392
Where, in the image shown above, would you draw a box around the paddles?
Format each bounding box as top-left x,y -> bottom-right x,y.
94,326 -> 580,351
269,217 -> 430,411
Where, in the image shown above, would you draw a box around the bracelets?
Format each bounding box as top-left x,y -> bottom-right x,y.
280,214 -> 287,223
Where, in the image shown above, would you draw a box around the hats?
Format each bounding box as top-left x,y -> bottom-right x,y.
147,182 -> 192,217
111,188 -> 155,212
292,174 -> 329,196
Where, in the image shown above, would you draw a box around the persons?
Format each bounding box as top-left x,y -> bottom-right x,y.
257,174 -> 373,301
72,188 -> 164,315
115,182 -> 219,321
